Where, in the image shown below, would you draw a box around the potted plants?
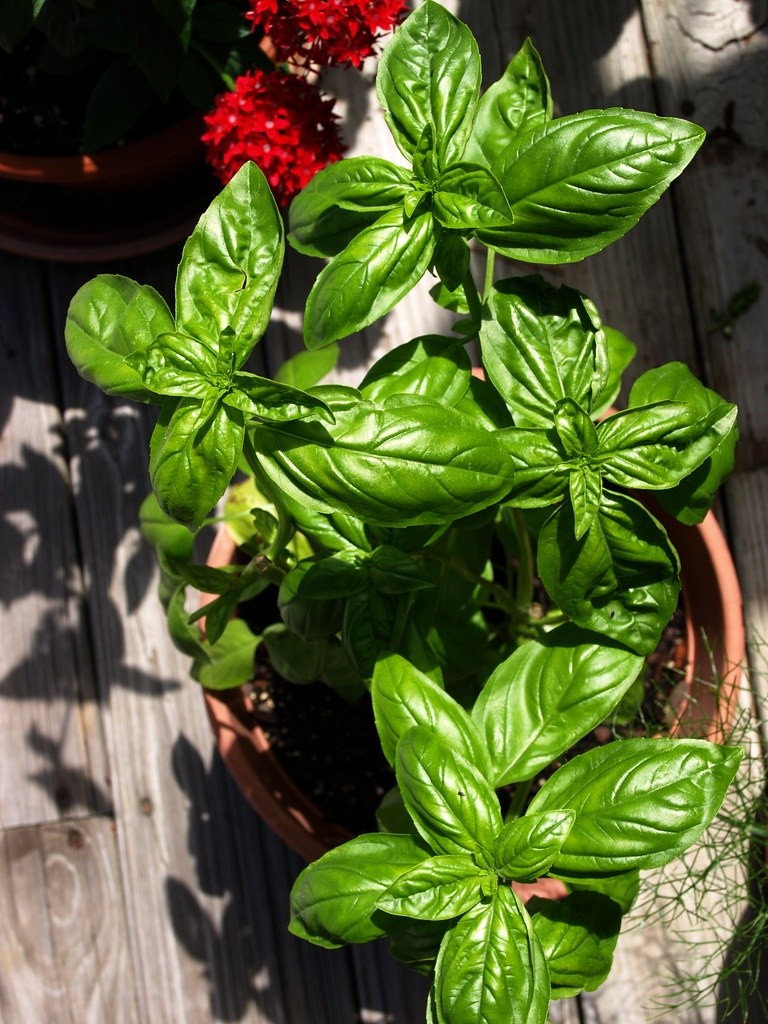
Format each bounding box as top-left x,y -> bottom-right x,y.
63,2 -> 747,1024
0,0 -> 407,265
